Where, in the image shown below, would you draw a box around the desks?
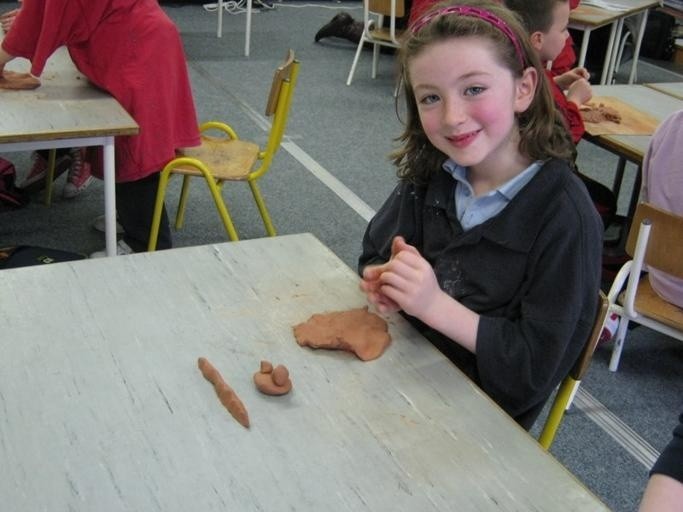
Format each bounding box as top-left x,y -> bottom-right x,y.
547,1 -> 683,266
0,233 -> 611,512
0,45 -> 138,257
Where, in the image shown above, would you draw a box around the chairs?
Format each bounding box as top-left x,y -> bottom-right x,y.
345,0 -> 405,97
218,1 -> 260,57
565,202 -> 682,412
148,49 -> 301,253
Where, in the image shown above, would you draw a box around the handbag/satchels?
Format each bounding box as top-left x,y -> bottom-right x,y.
0,244 -> 89,273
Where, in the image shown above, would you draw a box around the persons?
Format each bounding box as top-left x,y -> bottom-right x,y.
596,109 -> 683,345
1,0 -> 97,199
0,0 -> 204,258
500,0 -> 618,234
356,0 -> 606,433
314,9 -> 411,56
637,410 -> 683,511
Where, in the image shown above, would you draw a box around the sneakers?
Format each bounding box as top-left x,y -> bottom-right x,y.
91,239 -> 137,258
94,210 -> 126,233
21,148 -> 71,194
314,13 -> 353,42
64,144 -> 99,198
